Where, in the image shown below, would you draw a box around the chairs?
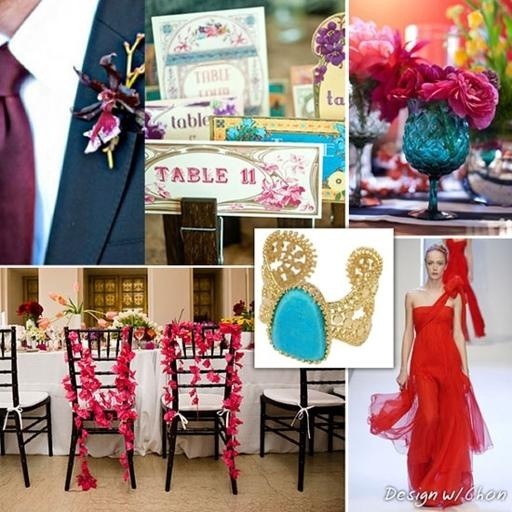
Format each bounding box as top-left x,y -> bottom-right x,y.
160,323 -> 239,494
0,325 -> 53,486
259,368 -> 345,491
63,326 -> 137,491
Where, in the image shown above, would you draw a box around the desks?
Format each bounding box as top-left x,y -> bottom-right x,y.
0,333 -> 346,459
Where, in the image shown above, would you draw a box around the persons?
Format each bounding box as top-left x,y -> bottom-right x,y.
366,243 -> 494,508
442,239 -> 474,284
0,0 -> 145,264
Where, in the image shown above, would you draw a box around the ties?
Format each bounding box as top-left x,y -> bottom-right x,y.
0,42 -> 34,265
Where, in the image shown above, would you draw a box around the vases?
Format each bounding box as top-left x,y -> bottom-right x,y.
132,337 -> 137,349
70,314 -> 81,330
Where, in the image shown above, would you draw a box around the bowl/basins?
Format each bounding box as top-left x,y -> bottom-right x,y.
223,331 -> 251,348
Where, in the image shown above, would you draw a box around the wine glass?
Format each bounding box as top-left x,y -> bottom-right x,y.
401,97 -> 470,220
135,326 -> 145,351
350,85 -> 390,191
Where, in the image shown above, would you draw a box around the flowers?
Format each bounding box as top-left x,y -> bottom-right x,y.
106,307 -> 163,338
349,15 -> 500,131
445,0 -> 512,96
35,278 -> 117,329
16,301 -> 44,325
221,299 -> 254,331
